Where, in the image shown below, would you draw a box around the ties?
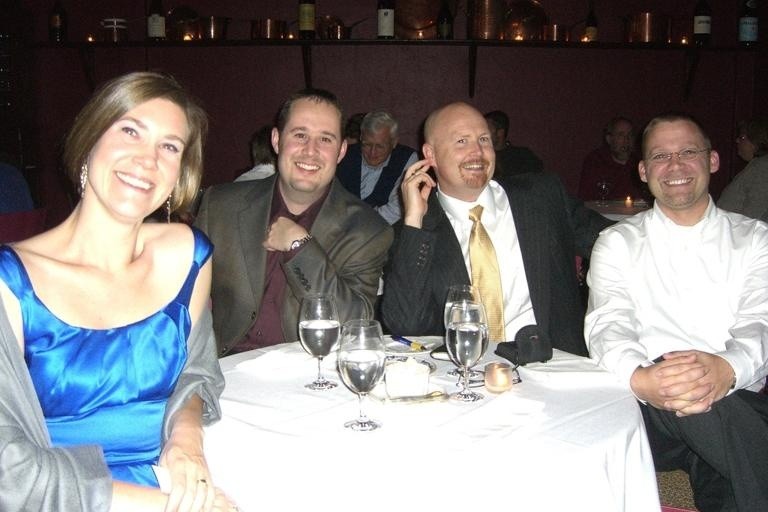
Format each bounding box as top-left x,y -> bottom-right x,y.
468,204 -> 506,344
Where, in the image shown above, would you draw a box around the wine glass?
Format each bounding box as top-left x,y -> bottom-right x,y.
443,284 -> 484,380
446,301 -> 489,403
297,291 -> 341,390
596,182 -> 609,207
337,318 -> 382,433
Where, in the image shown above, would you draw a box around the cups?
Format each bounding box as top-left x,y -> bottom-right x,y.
485,361 -> 513,393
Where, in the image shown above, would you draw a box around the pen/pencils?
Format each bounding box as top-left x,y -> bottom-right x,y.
390,334 -> 427,352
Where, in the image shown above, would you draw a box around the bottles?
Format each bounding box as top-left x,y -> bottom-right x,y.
46,1 -> 759,47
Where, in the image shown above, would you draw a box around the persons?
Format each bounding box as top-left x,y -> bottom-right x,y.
339,112 -> 420,224
482,111 -> 544,185
250,126 -> 280,166
717,117 -> 766,221
0,70 -> 237,511
1,163 -> 36,215
597,119 -> 651,198
192,86 -> 396,360
583,111 -> 768,512
379,100 -> 619,360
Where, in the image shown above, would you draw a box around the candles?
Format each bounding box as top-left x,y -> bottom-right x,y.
625,197 -> 634,208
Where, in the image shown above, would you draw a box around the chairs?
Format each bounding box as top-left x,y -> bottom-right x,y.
0,206 -> 48,244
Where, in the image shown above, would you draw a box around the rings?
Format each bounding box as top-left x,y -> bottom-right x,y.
197,478 -> 208,484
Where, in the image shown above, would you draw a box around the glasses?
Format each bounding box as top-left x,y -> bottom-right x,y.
643,147 -> 710,163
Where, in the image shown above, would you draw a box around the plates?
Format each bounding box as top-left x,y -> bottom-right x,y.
383,354 -> 438,379
630,201 -> 649,207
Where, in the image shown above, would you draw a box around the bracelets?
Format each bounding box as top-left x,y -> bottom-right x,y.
289,234 -> 312,250
